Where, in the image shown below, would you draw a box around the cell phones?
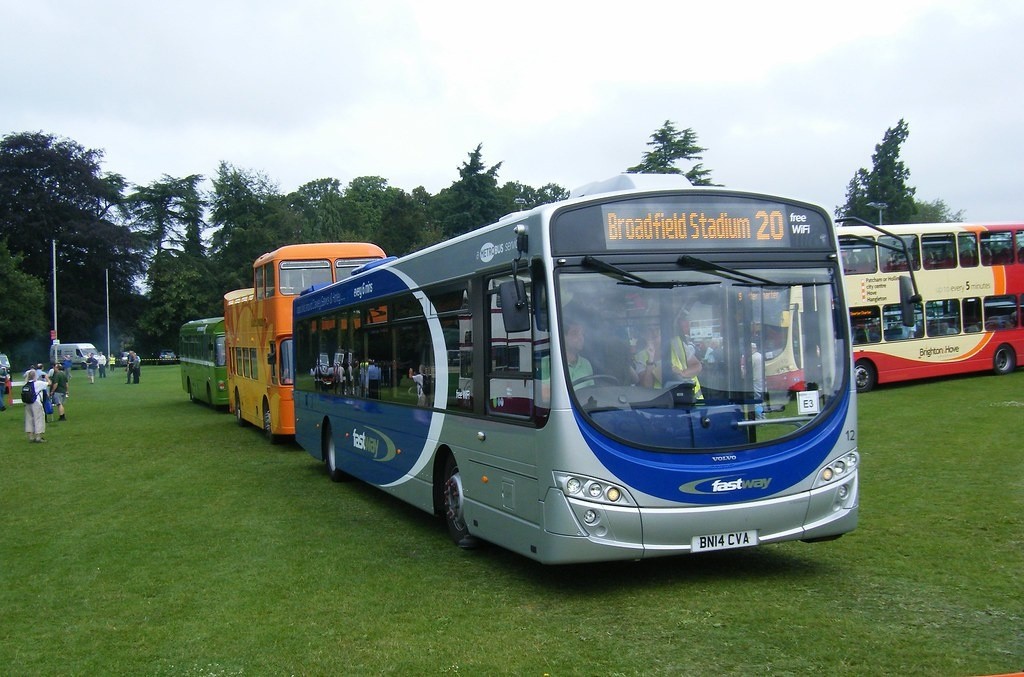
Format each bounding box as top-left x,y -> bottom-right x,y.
42,375 -> 45,378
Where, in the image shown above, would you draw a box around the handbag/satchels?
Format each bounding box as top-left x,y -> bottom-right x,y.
43,389 -> 54,414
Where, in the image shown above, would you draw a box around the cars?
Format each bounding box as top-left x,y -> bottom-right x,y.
120,351 -> 130,366
0,353 -> 12,381
149,348 -> 177,366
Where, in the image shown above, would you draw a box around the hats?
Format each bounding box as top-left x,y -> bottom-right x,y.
751,343 -> 757,348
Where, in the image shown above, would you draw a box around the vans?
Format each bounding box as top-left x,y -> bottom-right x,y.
49,343 -> 101,370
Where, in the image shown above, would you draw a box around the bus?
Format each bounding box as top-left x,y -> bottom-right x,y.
222,240 -> 387,445
833,217 -> 1024,392
290,170 -> 861,568
178,317 -> 229,409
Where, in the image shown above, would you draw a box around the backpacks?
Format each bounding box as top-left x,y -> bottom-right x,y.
416,374 -> 431,396
21,380 -> 41,404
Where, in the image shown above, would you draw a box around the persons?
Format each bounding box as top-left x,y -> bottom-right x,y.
64,355 -> 72,380
936,320 -> 958,335
125,351 -> 141,384
98,352 -> 106,378
409,365 -> 428,407
85,352 -> 98,384
22,363 -> 69,442
109,354 -> 115,372
0,366 -> 7,411
705,336 -> 767,427
541,316 -> 594,402
631,324 -> 705,405
891,324 -> 916,339
310,359 -> 390,400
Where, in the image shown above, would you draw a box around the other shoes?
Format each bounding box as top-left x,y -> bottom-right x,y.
28,437 -> 48,443
58,417 -> 66,421
1,407 -> 6,411
